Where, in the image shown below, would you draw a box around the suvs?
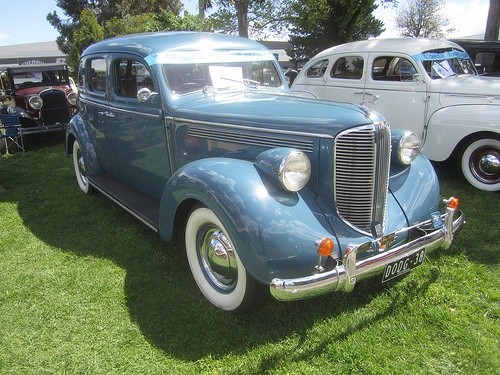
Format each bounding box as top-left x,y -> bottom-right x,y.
289,37 -> 500,193
0,63 -> 79,152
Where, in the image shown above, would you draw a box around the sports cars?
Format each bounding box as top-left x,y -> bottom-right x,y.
64,32 -> 465,314
